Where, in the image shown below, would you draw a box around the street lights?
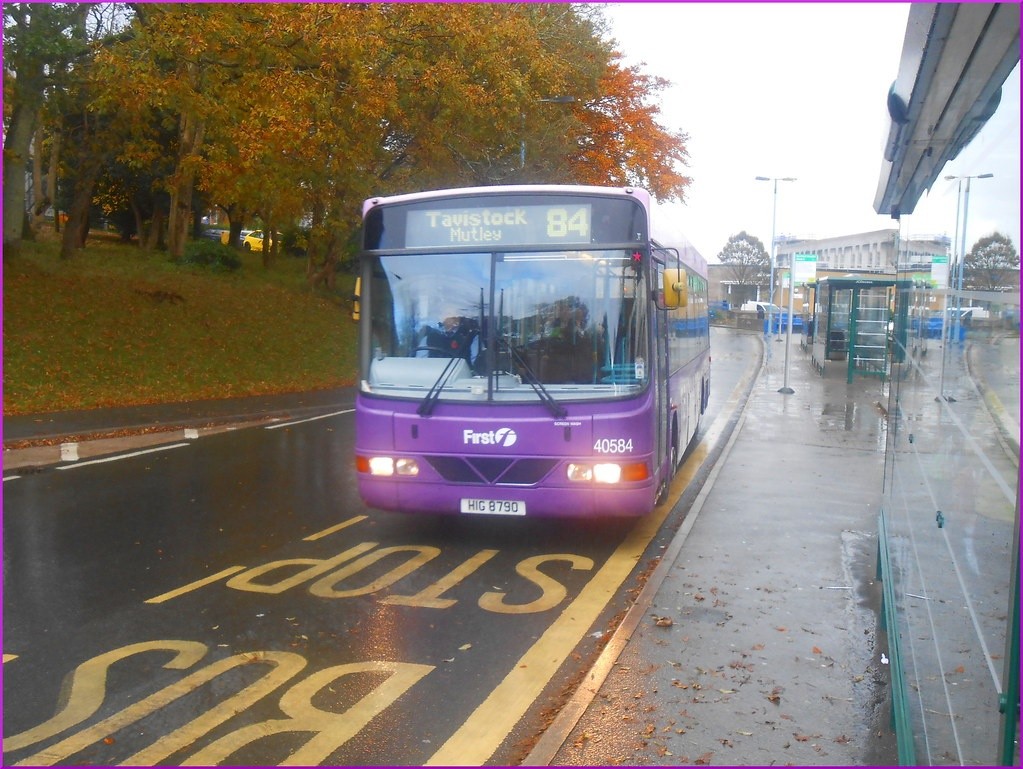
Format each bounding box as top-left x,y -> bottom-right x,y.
756,176 -> 798,337
945,173 -> 994,378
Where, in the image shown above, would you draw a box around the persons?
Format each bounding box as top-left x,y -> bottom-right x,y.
415,301 -> 479,370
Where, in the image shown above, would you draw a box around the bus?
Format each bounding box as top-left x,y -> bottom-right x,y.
327,183 -> 710,521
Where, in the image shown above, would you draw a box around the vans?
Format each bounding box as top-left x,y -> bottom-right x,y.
740,300 -> 781,313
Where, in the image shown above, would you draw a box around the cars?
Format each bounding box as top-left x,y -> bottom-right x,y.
200,228 -> 284,254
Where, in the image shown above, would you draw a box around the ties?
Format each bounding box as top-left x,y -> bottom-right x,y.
445,331 -> 453,338
